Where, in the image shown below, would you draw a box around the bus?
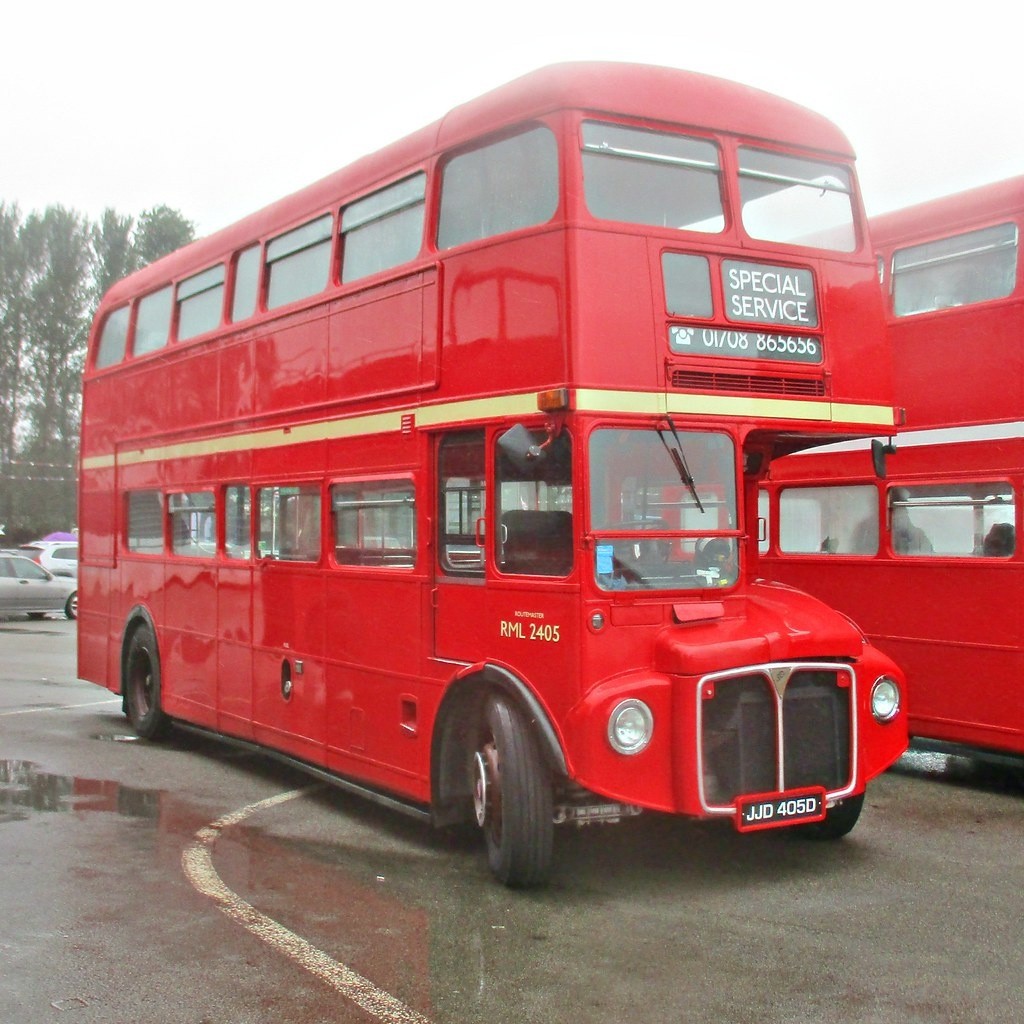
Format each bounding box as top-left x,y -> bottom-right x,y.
603,170 -> 1024,779
77,66 -> 914,887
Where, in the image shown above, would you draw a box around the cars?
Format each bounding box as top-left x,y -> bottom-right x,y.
16,541 -> 80,579
0,553 -> 77,620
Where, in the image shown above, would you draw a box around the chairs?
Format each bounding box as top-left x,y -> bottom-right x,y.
501,510 -> 573,577
